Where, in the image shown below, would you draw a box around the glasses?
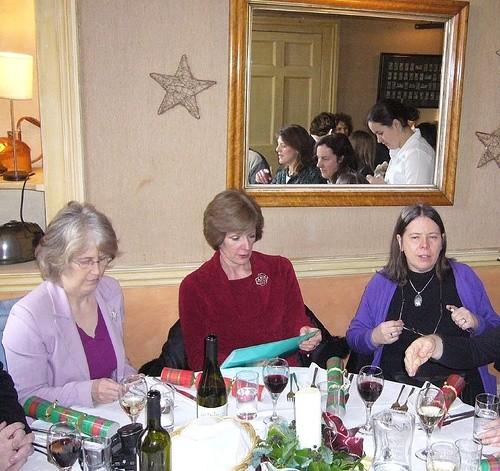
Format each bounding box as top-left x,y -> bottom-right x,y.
399,299 -> 443,337
71,255 -> 112,270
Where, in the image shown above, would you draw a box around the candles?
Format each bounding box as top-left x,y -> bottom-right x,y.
294,381 -> 322,451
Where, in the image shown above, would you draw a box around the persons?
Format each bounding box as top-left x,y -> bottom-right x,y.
1,201 -> 138,411
346,204 -> 500,445
0,361 -> 35,471
248,100 -> 437,185
179,189 -> 323,368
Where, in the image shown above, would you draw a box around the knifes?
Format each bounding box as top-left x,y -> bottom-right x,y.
417,410 -> 475,430
153,377 -> 196,402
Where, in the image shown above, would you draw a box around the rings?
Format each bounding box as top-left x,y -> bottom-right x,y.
463,318 -> 467,322
313,346 -> 315,349
112,396 -> 115,402
391,332 -> 393,338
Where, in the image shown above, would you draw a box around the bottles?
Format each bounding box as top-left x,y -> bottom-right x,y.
196,335 -> 228,419
136,390 -> 171,471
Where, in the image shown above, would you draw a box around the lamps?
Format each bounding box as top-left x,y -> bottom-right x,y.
0,54 -> 35,180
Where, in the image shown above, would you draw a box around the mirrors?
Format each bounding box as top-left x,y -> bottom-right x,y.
225,0 -> 470,206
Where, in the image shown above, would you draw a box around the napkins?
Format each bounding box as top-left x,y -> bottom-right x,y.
161,368 -> 266,403
22,396 -> 120,441
431,375 -> 465,428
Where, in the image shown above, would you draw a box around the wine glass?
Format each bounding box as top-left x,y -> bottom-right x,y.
415,388 -> 447,461
117,374 -> 148,424
357,366 -> 384,434
261,358 -> 290,424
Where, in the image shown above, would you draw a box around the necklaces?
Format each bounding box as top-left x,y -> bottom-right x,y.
406,271 -> 435,307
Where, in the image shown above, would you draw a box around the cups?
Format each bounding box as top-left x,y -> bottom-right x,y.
82,435 -> 112,471
473,393 -> 500,445
318,381 -> 346,421
236,370 -> 259,420
149,383 -> 174,433
455,438 -> 483,471
46,422 -> 82,471
426,441 -> 461,471
372,410 -> 415,471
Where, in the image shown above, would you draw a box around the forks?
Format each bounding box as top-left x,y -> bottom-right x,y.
287,373 -> 295,402
390,384 -> 415,412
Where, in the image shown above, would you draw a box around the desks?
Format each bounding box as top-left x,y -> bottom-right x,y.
19,367 -> 500,471
